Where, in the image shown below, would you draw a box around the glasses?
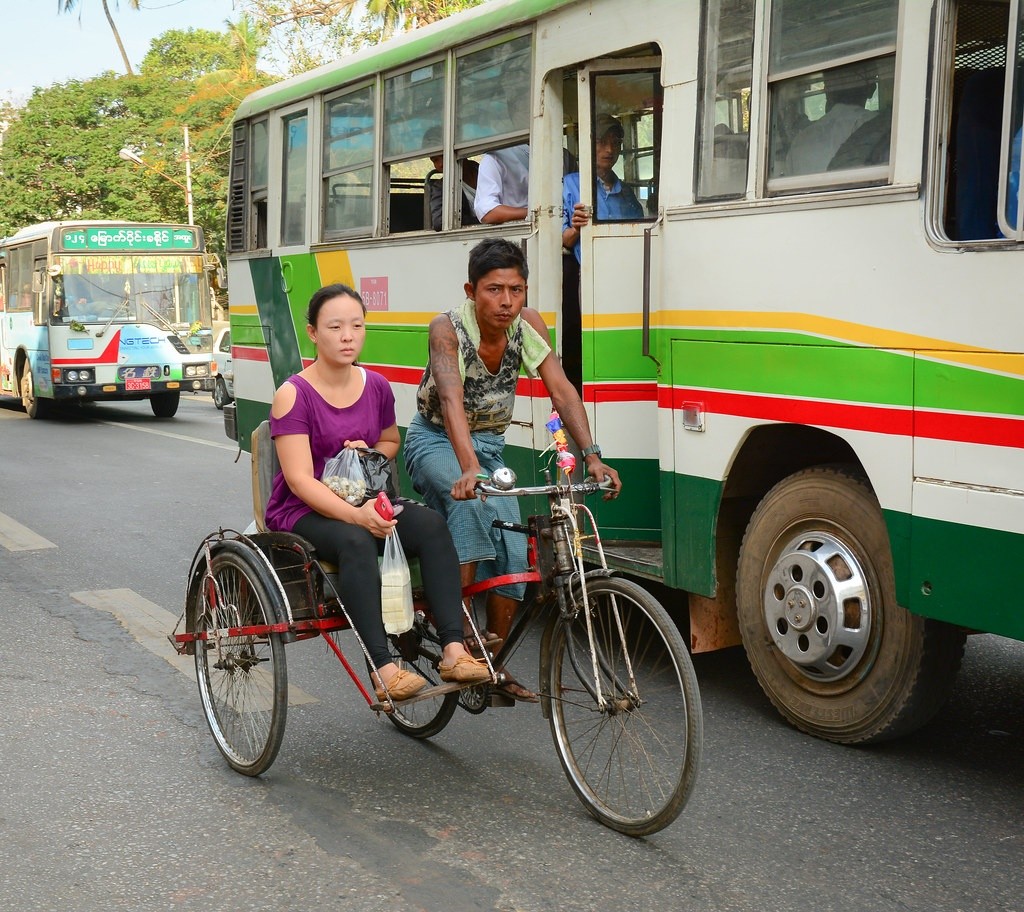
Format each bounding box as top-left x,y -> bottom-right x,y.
595,136 -> 623,145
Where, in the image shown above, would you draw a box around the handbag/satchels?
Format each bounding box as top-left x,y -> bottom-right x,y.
321,445 -> 366,506
356,448 -> 397,505
382,526 -> 414,635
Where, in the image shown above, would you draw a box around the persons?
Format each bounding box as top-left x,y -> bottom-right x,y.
63,276 -> 94,316
422,126 -> 479,231
403,239 -> 622,702
265,284 -> 488,698
769,31 -> 1024,239
715,123 -> 748,158
473,85 -> 578,224
562,115 -> 644,401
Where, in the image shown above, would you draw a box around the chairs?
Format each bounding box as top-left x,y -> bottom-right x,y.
251,421 -> 384,604
424,169 -> 444,229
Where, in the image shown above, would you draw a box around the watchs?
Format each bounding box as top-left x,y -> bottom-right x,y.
582,444 -> 602,462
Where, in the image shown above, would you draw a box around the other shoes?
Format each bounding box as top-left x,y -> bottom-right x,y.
376,660 -> 426,702
439,654 -> 489,682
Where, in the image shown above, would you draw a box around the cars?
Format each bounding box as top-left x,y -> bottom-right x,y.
211,328 -> 231,407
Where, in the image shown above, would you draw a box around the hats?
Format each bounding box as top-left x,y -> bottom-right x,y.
595,113 -> 624,138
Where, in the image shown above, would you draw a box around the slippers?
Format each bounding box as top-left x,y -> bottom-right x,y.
463,629 -> 503,650
487,679 -> 539,703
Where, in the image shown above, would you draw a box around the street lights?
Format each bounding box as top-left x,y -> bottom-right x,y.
118,147 -> 194,225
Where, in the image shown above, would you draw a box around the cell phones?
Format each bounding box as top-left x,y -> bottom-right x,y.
374,491 -> 394,522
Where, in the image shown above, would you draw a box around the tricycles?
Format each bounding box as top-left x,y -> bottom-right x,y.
167,411 -> 725,842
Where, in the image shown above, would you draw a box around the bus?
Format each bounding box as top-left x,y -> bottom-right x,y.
0,219 -> 214,416
221,0 -> 1023,757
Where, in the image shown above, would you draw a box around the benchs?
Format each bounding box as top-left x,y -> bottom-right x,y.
333,180 -> 424,232
